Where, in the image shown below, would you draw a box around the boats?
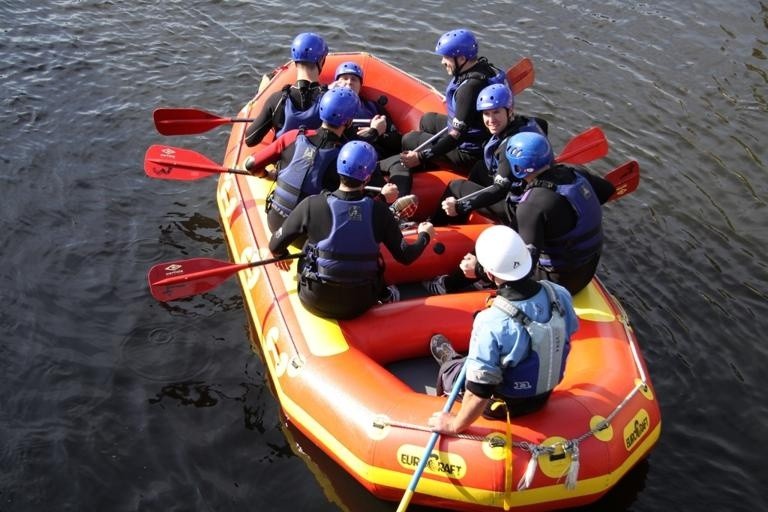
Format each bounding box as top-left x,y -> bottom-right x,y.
216,51 -> 661,512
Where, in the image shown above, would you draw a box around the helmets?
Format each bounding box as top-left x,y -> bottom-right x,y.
435,29 -> 478,59
334,61 -> 363,80
319,87 -> 361,125
291,33 -> 328,64
475,225 -> 532,282
476,83 -> 513,111
337,140 -> 378,181
505,131 -> 554,178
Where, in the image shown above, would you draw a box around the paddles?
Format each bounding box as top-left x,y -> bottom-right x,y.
145,145 -> 382,193
148,230 -> 418,301
456,127 -> 608,202
404,58 -> 535,155
604,160 -> 640,202
154,108 -> 373,136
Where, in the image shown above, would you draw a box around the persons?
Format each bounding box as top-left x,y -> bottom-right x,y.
420,131 -> 606,295
244,85 -> 401,251
332,62 -> 400,161
429,224 -> 578,437
245,33 -> 387,149
268,140 -> 436,320
419,83 -> 554,233
401,29 -> 517,173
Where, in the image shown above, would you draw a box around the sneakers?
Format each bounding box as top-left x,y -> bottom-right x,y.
378,284 -> 400,305
430,334 -> 464,367
421,275 -> 449,295
389,194 -> 419,225
